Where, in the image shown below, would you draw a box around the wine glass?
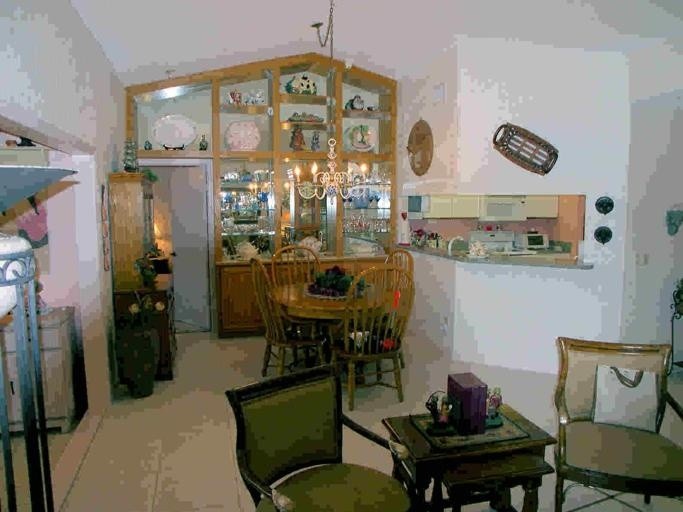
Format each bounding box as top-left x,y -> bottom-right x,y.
342,193 -> 347,208
374,191 -> 382,209
368,191 -> 374,208
347,193 -> 353,209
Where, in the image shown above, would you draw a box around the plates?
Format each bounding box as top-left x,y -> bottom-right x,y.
150,113 -> 198,149
465,253 -> 490,259
303,281 -> 371,300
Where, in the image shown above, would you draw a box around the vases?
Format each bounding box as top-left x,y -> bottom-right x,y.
115,325 -> 162,399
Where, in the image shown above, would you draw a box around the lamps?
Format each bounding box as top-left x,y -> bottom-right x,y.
291,4 -> 370,202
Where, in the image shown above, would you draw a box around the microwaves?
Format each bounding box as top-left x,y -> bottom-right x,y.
515,232 -> 550,250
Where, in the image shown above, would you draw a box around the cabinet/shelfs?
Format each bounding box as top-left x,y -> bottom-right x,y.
107,172 -> 176,383
123,54 -> 397,337
0,305 -> 80,434
526,195 -> 560,218
423,193 -> 480,218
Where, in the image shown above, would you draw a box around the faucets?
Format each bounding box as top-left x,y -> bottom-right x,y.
448,235 -> 464,256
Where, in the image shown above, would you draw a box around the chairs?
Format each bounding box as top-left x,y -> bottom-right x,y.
224,362 -> 415,512
548,336 -> 683,512
250,245 -> 416,410
